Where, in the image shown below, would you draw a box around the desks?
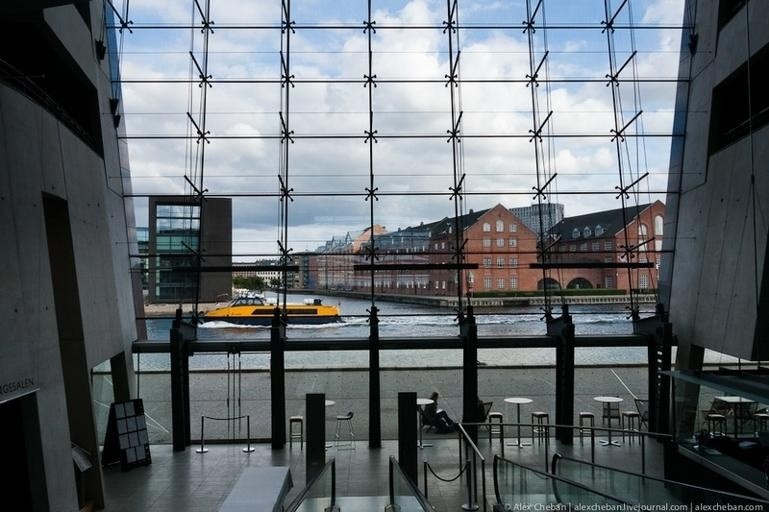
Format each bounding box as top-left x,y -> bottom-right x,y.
416,398 -> 435,449
503,398 -> 534,448
713,395 -> 758,415
593,397 -> 624,447
325,399 -> 336,406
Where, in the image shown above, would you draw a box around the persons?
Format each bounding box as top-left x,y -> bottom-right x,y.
423,389 -> 459,433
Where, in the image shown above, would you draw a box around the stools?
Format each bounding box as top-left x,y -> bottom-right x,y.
707,413 -> 769,436
579,411 -> 641,447
290,416 -> 304,450
488,412 -> 549,445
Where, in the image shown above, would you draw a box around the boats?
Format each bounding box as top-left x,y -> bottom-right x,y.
203,292 -> 341,326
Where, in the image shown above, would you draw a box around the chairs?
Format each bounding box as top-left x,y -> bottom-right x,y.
334,411 -> 354,450
603,396 -> 620,426
634,398 -> 649,431
479,402 -> 493,431
